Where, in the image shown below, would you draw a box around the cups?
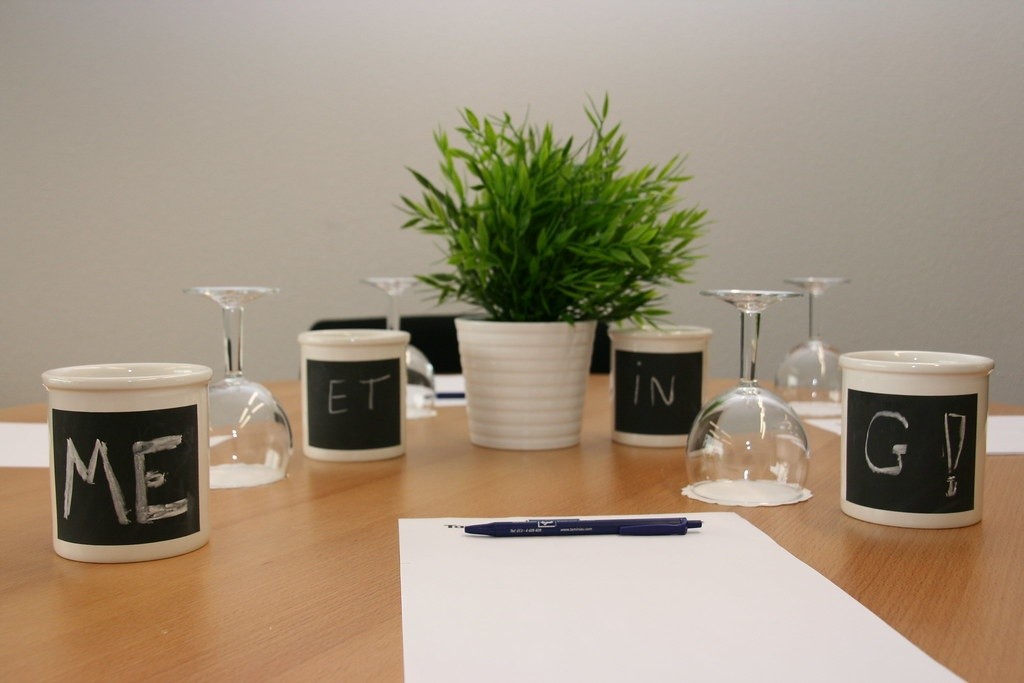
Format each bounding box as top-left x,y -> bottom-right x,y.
298,330 -> 411,462
608,326 -> 714,448
838,350 -> 996,528
40,362 -> 214,563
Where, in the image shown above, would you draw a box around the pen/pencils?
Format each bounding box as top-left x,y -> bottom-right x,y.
462,516 -> 703,537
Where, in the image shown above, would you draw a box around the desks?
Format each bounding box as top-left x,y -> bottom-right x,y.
0,371 -> 1024,683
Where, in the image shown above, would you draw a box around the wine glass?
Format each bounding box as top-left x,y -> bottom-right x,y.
194,286 -> 293,489
359,277 -> 438,420
776,275 -> 851,418
686,289 -> 808,505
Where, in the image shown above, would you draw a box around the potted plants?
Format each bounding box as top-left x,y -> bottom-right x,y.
392,93 -> 717,451
